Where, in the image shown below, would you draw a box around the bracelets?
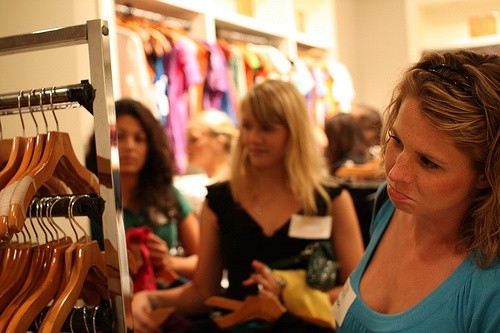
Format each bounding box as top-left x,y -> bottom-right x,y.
145,291 -> 160,308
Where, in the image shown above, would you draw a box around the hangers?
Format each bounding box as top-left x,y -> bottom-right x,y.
115,3 -> 330,74
0,86 -> 134,333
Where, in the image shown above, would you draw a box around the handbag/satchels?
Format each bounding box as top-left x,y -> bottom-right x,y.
306,239 -> 338,292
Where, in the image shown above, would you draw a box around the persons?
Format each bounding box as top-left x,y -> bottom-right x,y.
185,109 -> 242,288
331,50 -> 500,333
131,80 -> 365,333
85,99 -> 201,333
321,106 -> 386,176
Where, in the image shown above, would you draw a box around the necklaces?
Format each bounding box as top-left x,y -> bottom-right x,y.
247,172 -> 284,215
